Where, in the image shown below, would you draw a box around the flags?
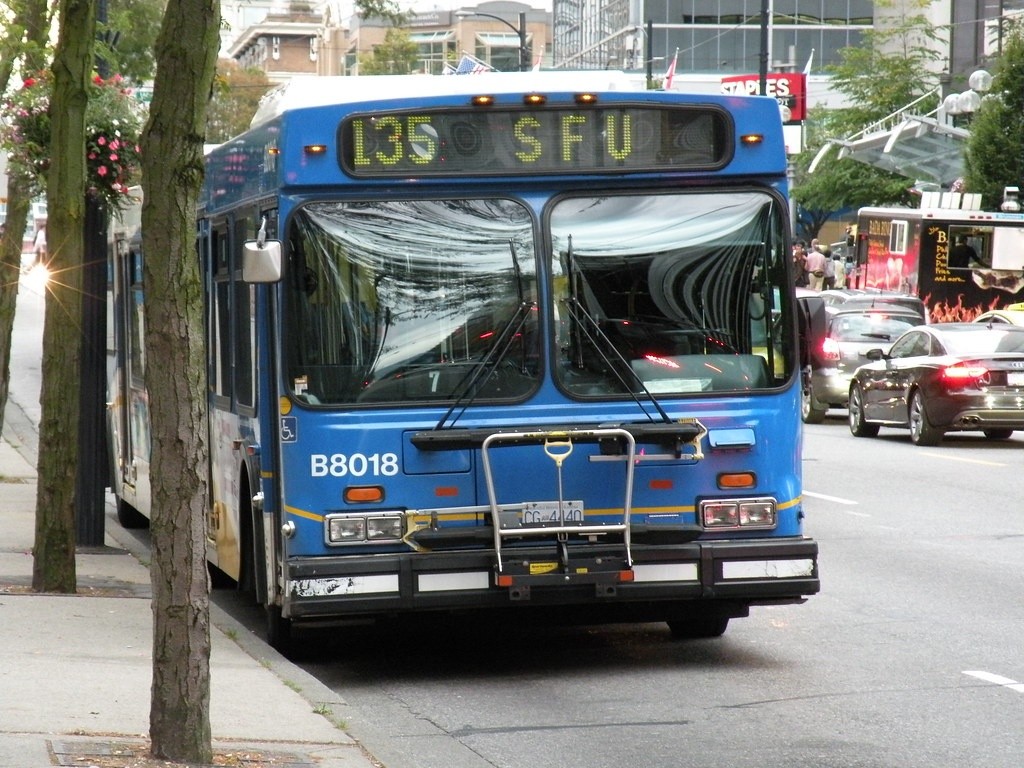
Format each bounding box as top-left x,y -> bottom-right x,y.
440,66 -> 457,76
662,55 -> 678,90
801,51 -> 813,92
456,55 -> 492,75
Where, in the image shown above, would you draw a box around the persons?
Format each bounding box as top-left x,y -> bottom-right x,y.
25,222 -> 48,275
948,235 -> 992,270
790,234 -> 858,293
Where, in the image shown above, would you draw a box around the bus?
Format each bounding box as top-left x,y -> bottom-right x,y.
845,208 -> 1023,321
87,70 -> 821,648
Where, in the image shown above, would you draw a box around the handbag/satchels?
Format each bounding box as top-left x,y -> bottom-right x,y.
813,268 -> 824,278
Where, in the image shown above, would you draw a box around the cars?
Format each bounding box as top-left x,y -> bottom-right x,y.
800,303 -> 924,424
971,302 -> 1023,328
815,290 -> 927,324
848,313 -> 1024,445
748,284 -> 818,378
